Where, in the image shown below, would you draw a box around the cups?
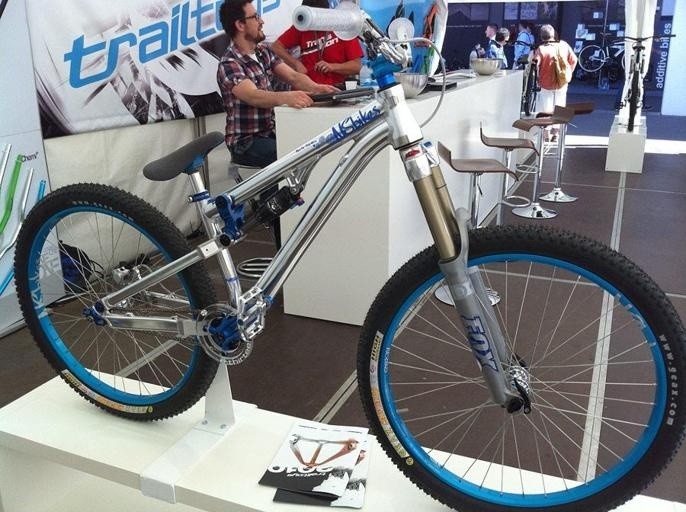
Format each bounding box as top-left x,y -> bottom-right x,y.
238,14 -> 259,23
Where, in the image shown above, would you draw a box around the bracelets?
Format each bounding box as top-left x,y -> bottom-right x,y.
471,57 -> 503,76
393,72 -> 429,100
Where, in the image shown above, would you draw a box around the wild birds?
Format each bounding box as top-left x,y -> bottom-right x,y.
310,85 -> 375,103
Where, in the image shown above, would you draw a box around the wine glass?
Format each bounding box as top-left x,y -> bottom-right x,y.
322,67 -> 326,70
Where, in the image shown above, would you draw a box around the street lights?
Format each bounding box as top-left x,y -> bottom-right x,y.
553,46 -> 571,89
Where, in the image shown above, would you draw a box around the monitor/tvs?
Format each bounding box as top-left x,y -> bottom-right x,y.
271,434 -> 375,510
259,419 -> 371,498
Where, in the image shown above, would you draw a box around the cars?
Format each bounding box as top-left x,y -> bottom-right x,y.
344,80 -> 357,90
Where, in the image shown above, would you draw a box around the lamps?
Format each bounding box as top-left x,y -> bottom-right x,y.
433,102 -> 597,308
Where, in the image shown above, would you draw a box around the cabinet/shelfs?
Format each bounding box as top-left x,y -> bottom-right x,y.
360,57 -> 378,100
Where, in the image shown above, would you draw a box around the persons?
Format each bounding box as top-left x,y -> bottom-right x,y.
469,19 -> 536,71
271,0 -> 365,95
216,0 -> 344,254
531,23 -> 579,145
